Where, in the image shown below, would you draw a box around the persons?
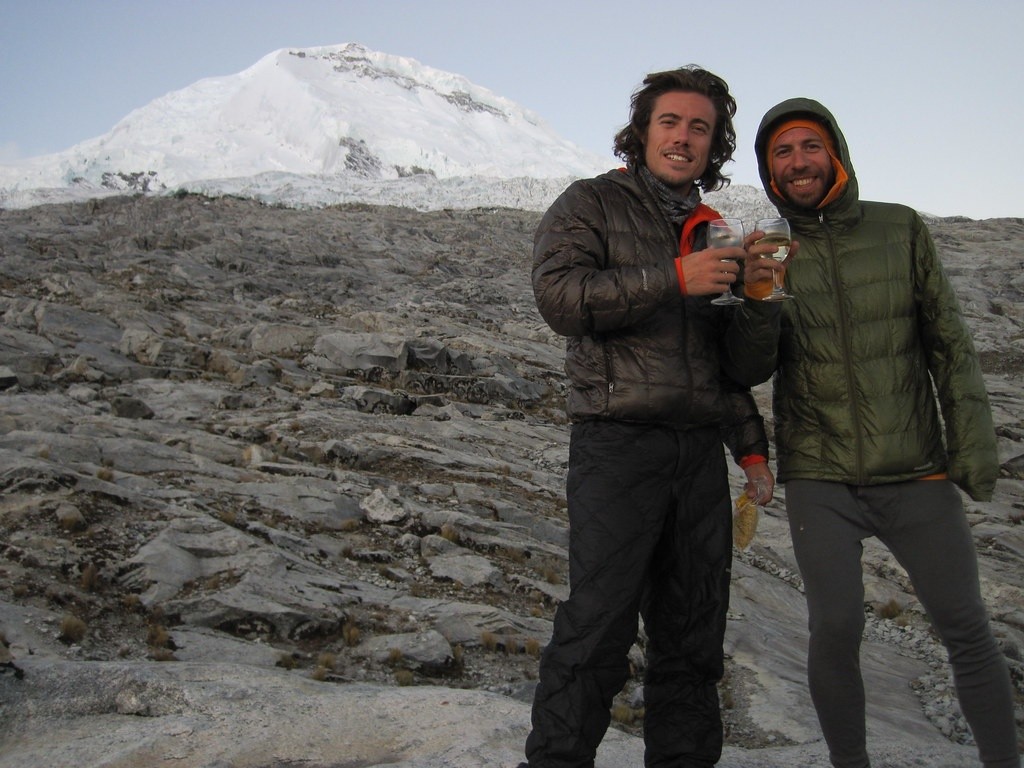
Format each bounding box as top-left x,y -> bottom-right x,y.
518,71 -> 775,768
732,97 -> 1017,767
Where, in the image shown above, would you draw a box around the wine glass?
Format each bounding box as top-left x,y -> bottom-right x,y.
754,218 -> 794,302
705,219 -> 745,305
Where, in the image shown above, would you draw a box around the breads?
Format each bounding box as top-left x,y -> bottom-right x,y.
731,495 -> 760,553
